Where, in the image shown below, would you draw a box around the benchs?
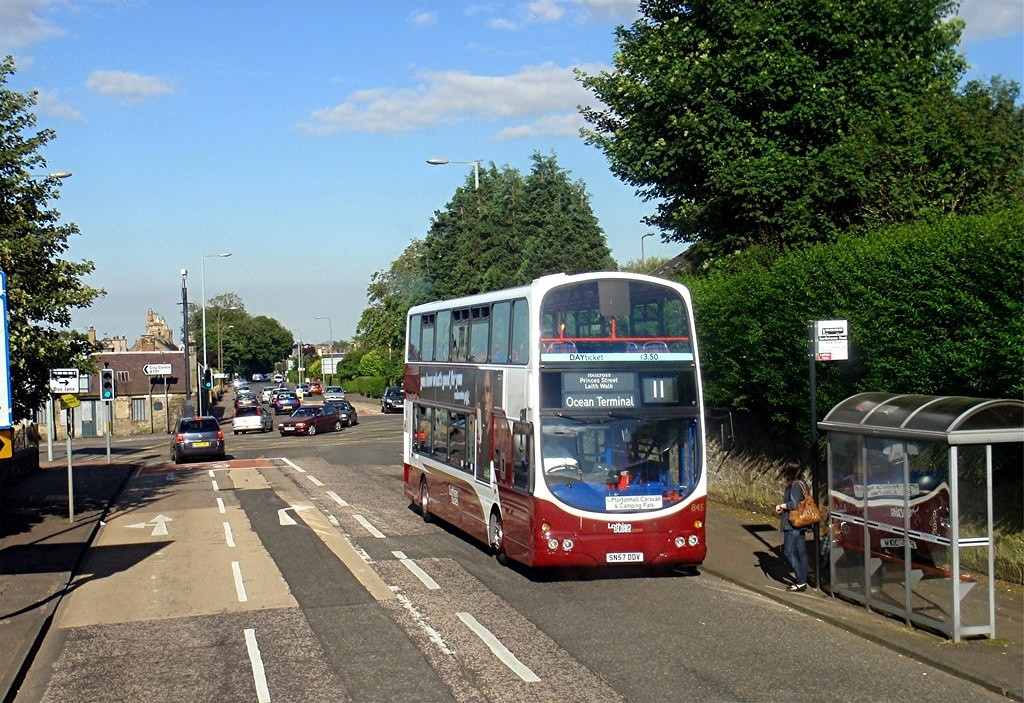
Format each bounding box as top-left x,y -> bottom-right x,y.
843,543 -> 977,592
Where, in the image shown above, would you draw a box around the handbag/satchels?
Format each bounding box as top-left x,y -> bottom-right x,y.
787,479 -> 821,527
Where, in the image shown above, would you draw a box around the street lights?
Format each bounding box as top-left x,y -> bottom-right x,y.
289,328 -> 305,383
220,326 -> 235,388
641,232 -> 655,271
316,317 -> 333,386
425,157 -> 484,272
217,307 -> 237,392
201,252 -> 233,416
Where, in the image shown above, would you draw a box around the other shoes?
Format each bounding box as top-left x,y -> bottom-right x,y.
786,582 -> 807,592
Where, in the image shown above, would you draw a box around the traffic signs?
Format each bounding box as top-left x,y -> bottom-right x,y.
48,368 -> 80,394
141,364 -> 173,375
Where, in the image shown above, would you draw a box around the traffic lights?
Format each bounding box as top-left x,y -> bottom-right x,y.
100,369 -> 115,402
204,369 -> 213,389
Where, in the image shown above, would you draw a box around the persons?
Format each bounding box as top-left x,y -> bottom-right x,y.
477,370 -> 492,478
543,435 -> 578,473
776,459 -> 810,591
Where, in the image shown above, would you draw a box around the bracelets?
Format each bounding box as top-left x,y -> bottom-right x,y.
779,504 -> 782,509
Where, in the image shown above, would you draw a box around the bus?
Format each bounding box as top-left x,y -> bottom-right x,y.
403,271 -> 737,568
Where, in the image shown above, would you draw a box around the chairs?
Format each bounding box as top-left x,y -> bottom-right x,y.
541,341 -> 579,353
623,341 -> 670,353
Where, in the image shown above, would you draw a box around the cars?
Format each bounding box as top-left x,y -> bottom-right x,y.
166,415 -> 226,465
379,387 -> 404,414
320,400 -> 358,428
231,404 -> 274,435
232,372 -> 347,415
277,404 -> 343,437
